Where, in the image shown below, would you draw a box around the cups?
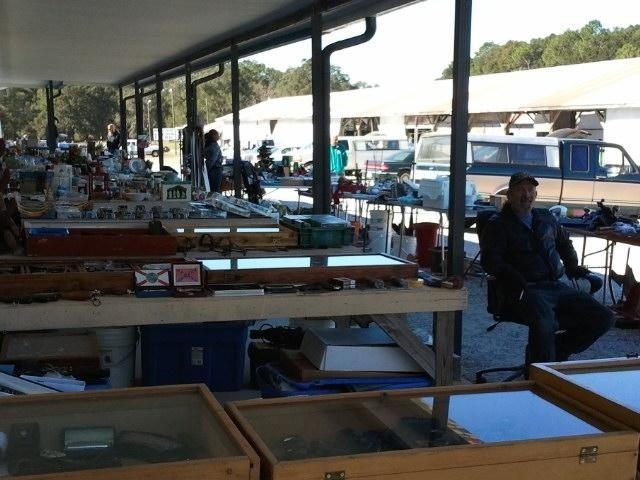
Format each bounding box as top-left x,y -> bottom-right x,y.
151,223 -> 161,235
63,426 -> 116,458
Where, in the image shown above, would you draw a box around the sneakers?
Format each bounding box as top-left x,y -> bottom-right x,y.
554,332 -> 571,361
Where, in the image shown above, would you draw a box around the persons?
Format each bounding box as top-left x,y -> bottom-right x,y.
107,123 -> 120,155
204,129 -> 224,194
479,171 -> 613,378
330,134 -> 348,174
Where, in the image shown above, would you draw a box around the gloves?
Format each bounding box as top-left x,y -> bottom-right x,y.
584,274 -> 605,294
507,275 -> 529,305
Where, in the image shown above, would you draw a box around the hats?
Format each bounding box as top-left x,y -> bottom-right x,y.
510,171 -> 538,189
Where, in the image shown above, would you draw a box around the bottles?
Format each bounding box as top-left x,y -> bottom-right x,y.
168,187 -> 180,198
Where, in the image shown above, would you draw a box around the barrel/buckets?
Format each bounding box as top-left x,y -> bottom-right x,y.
414,222 -> 441,267
368,210 -> 392,253
92,328 -> 137,388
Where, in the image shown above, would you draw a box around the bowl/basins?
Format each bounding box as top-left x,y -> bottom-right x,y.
126,193 -> 147,202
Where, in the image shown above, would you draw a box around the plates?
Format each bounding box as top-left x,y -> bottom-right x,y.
128,158 -> 145,174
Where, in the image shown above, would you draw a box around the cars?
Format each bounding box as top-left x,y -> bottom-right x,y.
120,139 -> 171,160
